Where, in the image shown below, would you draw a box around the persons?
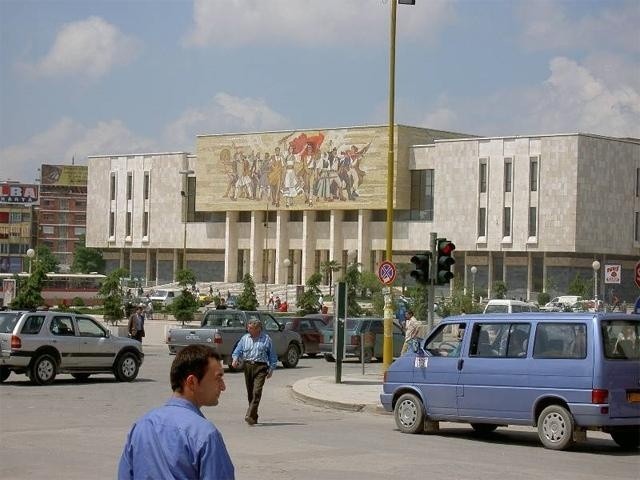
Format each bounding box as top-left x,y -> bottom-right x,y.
317,294 -> 325,311
126,306 -> 146,343
266,291 -> 274,306
221,139 -> 372,209
399,309 -> 421,355
208,284 -> 214,297
274,296 -> 282,312
226,290 -> 231,300
611,295 -> 621,310
434,337 -> 462,357
117,343 -> 235,479
457,309 -> 467,341
216,298 -> 227,310
507,328 -> 528,357
230,317 -> 277,426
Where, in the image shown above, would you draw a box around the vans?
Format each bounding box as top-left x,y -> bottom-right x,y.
382,313 -> 639,451
478,298 -> 541,330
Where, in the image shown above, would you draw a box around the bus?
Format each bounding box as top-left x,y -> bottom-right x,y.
0,269 -> 108,310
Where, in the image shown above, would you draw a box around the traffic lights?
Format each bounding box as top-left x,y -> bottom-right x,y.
437,237 -> 456,286
409,254 -> 429,284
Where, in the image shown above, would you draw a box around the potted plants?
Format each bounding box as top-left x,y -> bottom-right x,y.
164,268 -> 200,344
101,269 -> 130,337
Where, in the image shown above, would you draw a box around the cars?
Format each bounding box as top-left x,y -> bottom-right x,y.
269,308 -> 407,364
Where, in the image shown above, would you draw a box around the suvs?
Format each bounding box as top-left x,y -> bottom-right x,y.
1,306 -> 143,385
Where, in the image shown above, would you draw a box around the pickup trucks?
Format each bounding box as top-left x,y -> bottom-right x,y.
162,308 -> 305,375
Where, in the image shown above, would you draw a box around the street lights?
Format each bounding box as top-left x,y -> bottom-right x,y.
591,259 -> 600,311
382,0 -> 420,366
27,247 -> 36,284
283,258 -> 292,312
469,264 -> 478,312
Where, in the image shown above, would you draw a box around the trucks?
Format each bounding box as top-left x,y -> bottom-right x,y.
118,276 -> 182,319
537,293 -> 607,316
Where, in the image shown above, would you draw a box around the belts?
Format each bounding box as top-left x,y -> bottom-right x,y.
243,360 -> 267,366
